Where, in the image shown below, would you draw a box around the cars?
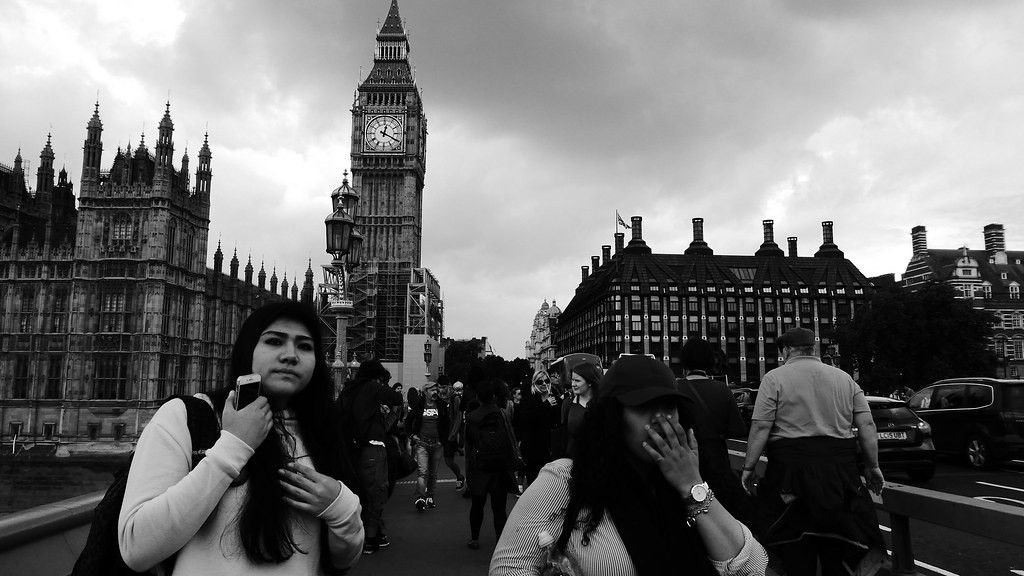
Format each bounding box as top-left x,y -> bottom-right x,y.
848,395 -> 938,484
732,386 -> 758,433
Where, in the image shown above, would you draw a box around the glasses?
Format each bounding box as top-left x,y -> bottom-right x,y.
536,379 -> 550,385
516,393 -> 521,395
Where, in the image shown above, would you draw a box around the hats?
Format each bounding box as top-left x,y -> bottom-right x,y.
775,327 -> 816,347
422,382 -> 436,391
599,355 -> 697,407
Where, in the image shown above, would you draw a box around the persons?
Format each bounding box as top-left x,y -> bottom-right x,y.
118,299 -> 366,576
741,328 -> 885,576
865,385 -> 934,409
483,354 -> 768,576
336,338 -> 744,555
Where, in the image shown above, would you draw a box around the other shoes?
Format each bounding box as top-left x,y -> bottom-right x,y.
363,542 -> 378,554
455,475 -> 466,492
414,496 -> 426,513
426,495 -> 436,508
467,539 -> 479,550
366,533 -> 390,547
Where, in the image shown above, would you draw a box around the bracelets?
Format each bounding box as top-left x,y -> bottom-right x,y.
742,465 -> 756,472
686,489 -> 714,528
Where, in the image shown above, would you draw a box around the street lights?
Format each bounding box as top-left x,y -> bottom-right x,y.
423,337 -> 432,383
996,336 -> 1009,379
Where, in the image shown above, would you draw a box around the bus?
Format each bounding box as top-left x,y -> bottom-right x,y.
548,353 -> 603,387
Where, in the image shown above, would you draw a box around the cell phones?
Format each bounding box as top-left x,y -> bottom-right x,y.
233,373 -> 261,411
549,394 -> 556,404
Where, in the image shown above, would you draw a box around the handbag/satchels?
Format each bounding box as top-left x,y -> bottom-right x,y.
395,449 -> 418,479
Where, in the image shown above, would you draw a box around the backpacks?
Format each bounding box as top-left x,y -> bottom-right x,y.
69,393 -> 219,576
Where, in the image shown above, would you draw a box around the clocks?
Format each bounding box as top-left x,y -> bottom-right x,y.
365,115 -> 403,151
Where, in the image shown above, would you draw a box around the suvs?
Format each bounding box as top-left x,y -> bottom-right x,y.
906,377 -> 1024,470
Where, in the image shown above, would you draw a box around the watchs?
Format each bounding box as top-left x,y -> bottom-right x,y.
677,481 -> 710,505
